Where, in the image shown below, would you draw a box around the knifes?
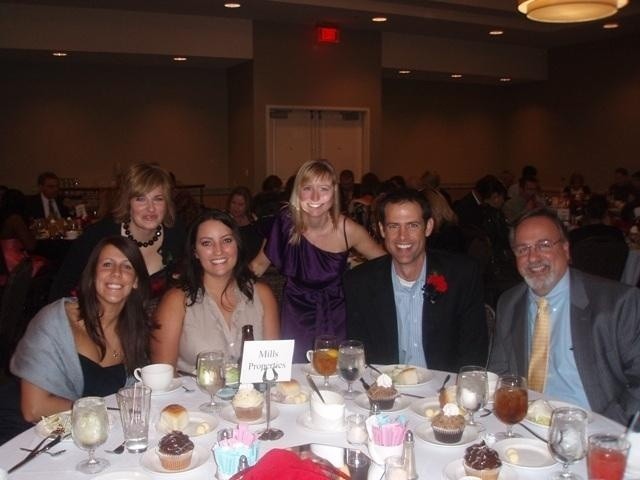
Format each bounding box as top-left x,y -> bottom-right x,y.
7,434 -> 66,473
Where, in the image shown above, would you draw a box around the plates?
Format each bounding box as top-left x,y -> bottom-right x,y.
523,398 -> 594,429
355,391 -> 411,414
218,400 -> 281,425
372,362 -> 435,386
153,411 -> 219,438
35,412 -> 72,441
141,377 -> 183,395
296,411 -> 353,432
312,337 -> 341,390
621,429 -> 640,477
411,394 -> 469,419
493,438 -> 558,468
264,386 -> 310,404
140,444 -> 208,475
414,419 -> 479,447
441,458 -> 517,480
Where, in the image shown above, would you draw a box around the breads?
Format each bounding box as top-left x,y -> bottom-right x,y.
439,385 -> 457,408
160,404 -> 189,429
277,379 -> 301,397
396,368 -> 418,385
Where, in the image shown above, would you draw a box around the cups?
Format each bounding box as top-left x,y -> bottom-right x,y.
472,368 -> 500,401
587,431 -> 631,480
116,386 -> 148,452
132,363 -> 174,392
311,390 -> 345,422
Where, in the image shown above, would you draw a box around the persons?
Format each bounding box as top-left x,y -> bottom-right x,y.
0,158 -> 640,433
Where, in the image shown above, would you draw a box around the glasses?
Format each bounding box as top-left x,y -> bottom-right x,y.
513,240 -> 561,257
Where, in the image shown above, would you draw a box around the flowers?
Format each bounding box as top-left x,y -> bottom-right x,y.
421,273 -> 448,304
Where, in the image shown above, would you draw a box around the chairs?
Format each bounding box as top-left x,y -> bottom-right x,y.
139,297 -> 163,325
256,266 -> 288,317
484,305 -> 496,371
0,257 -> 33,368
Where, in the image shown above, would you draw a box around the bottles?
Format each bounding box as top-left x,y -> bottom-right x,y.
30,216 -> 84,240
238,324 -> 255,384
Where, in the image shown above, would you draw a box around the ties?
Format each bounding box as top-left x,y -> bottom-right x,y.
528,298 -> 549,394
49,199 -> 58,218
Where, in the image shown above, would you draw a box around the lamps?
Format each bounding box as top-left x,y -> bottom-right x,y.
514,0 -> 631,24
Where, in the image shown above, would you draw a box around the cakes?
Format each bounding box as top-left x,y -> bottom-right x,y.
366,374 -> 398,410
155,431 -> 194,470
462,440 -> 502,480
232,383 -> 264,422
431,403 -> 465,444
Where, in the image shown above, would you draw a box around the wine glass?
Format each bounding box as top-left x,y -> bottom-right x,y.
457,365 -> 489,435
493,370 -> 528,441
71,397 -> 111,474
193,349 -> 228,414
335,340 -> 362,401
548,404 -> 590,480
